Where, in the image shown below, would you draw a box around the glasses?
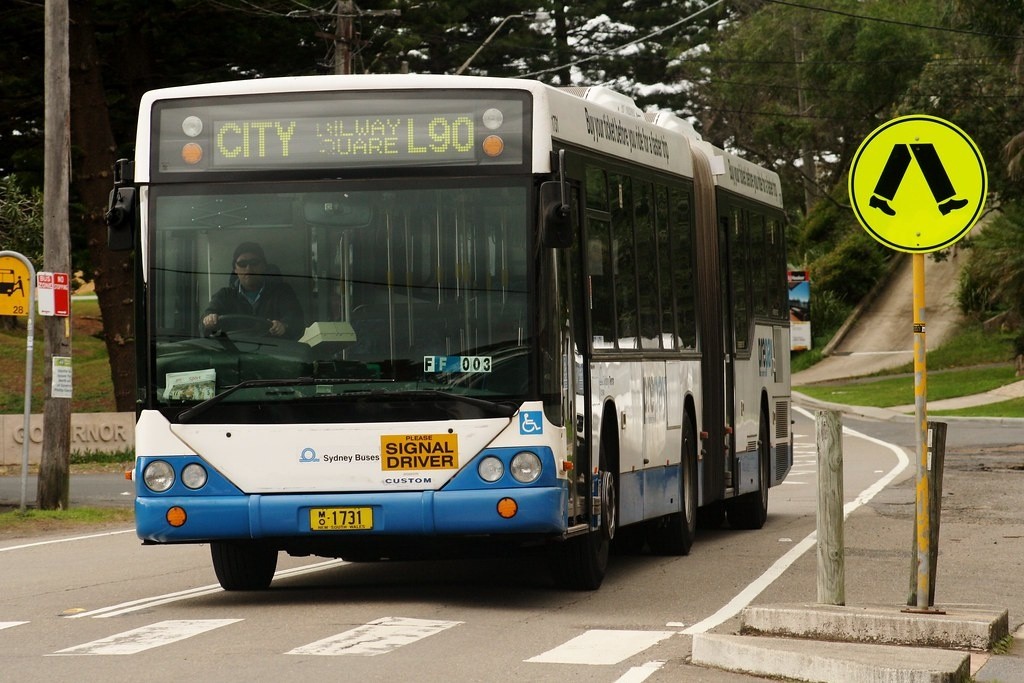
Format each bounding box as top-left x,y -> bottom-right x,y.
235,257 -> 265,268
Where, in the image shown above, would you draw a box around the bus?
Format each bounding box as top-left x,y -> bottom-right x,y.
102,71 -> 798,600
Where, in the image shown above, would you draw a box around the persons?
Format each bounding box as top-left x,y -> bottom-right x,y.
200,242 -> 305,340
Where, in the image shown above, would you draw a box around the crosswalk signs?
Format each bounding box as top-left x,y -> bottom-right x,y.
851,114 -> 986,254
